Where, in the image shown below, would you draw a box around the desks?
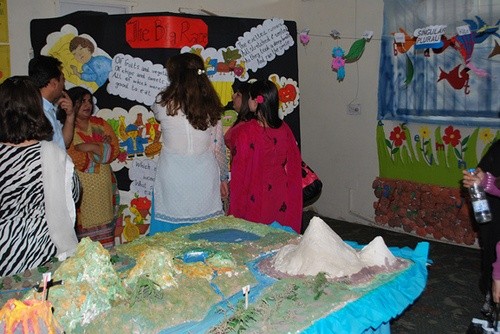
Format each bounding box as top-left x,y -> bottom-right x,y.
0,215 -> 433,334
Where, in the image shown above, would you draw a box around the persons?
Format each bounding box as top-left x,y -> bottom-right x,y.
27,54 -> 75,152
0,75 -> 80,279
149,52 -> 226,236
457,137 -> 500,334
228,81 -> 257,128
225,80 -> 302,234
56,85 -> 120,250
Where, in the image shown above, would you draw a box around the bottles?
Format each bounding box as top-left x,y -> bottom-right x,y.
467,168 -> 492,223
